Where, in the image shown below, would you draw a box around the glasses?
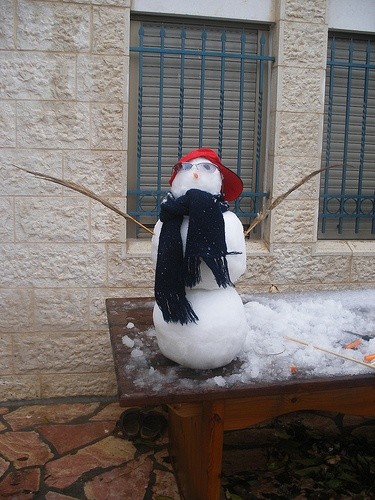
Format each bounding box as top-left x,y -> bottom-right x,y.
172,162 -> 219,174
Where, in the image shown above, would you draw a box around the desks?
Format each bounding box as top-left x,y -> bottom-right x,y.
106,285 -> 374,499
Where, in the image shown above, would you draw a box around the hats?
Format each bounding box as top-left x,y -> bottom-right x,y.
168,148 -> 243,202
